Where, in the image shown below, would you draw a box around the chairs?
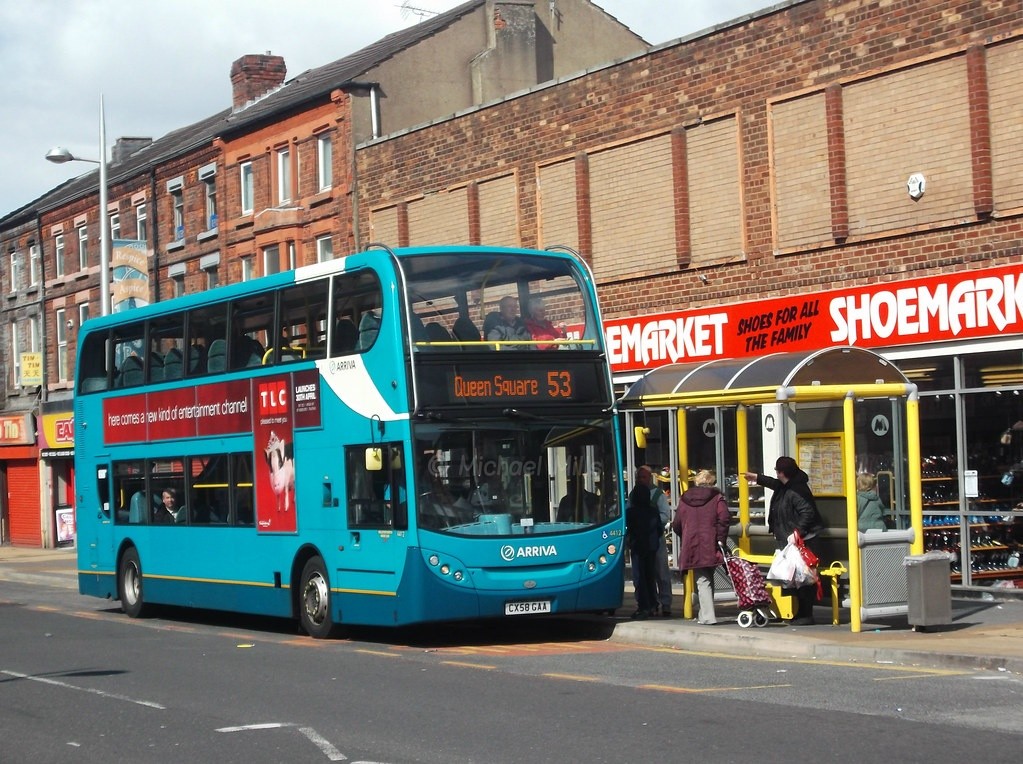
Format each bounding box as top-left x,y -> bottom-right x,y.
81,311 -> 520,394
130,488 -> 254,522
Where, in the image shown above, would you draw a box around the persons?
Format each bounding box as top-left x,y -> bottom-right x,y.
740,457 -> 825,625
557,475 -> 601,525
625,464 -> 672,618
368,472 -> 407,517
524,299 -> 568,349
856,473 -> 886,534
484,296 -> 538,351
153,487 -> 181,524
672,470 -> 730,624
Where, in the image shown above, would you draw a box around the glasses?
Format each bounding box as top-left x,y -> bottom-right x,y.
774,467 -> 777,470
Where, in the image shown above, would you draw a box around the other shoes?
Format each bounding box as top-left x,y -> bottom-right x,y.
783,615 -> 814,625
652,610 -> 672,617
842,599 -> 850,608
632,609 -> 655,620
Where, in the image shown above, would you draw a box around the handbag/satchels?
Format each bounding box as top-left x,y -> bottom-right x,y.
766,528 -> 823,601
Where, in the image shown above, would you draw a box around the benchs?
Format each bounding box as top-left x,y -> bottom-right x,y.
731,548 -> 847,625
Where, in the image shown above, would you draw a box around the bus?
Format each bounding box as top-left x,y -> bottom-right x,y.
71,242 -> 652,639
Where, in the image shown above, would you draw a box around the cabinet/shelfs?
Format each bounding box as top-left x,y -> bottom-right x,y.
658,473 -> 1023,581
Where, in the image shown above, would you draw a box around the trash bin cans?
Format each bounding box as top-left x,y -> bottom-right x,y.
905,557 -> 953,631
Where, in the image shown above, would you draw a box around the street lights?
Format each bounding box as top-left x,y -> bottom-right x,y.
45,93 -> 112,388
